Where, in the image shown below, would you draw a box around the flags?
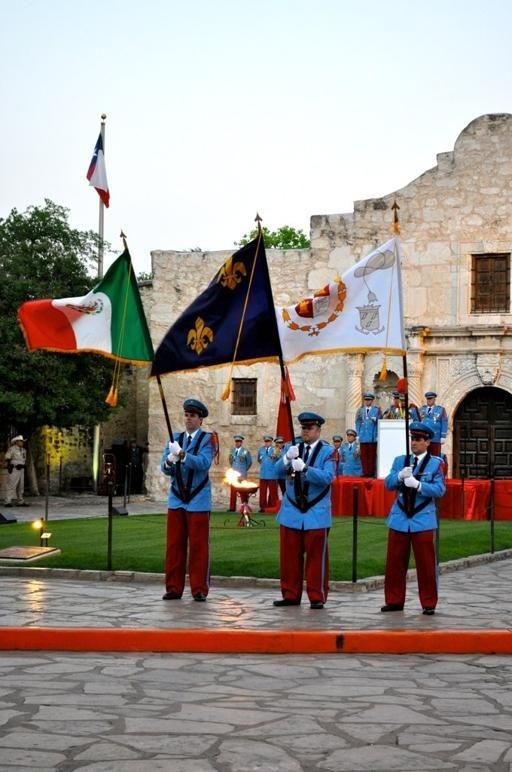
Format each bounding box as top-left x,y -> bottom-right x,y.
145,231 -> 282,383
85,131 -> 110,208
274,236 -> 407,367
15,246 -> 154,366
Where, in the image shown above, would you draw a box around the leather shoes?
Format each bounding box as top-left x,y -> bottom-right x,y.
381,605 -> 404,611
310,600 -> 324,609
423,608 -> 435,615
162,592 -> 182,599
4,503 -> 30,507
273,599 -> 300,606
195,592 -> 206,601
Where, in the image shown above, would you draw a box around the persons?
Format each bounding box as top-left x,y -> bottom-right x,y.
272,410 -> 339,610
159,399 -> 216,602
2,434 -> 31,508
124,438 -> 149,495
332,436 -> 343,475
339,429 -> 363,477
381,419 -> 447,616
382,390 -> 421,422
354,392 -> 383,478
226,432 -> 285,510
416,391 -> 449,458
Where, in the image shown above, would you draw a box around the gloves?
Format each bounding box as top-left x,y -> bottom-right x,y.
167,440 -> 182,465
440,438 -> 445,444
399,464 -> 419,489
355,436 -> 360,445
286,446 -> 306,472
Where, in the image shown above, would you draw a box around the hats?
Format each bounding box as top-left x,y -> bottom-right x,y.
409,422 -> 434,439
11,435 -> 28,445
362,391 -> 437,400
298,412 -> 325,428
234,430 -> 357,443
183,399 -> 208,417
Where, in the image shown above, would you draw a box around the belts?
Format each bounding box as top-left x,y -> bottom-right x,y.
12,464 -> 24,468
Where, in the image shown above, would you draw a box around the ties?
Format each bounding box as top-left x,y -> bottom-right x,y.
236,450 -> 238,456
367,409 -> 369,413
304,446 -> 311,463
413,457 -> 418,472
266,446 -> 268,454
428,408 -> 431,412
187,436 -> 192,447
349,447 -> 351,451
